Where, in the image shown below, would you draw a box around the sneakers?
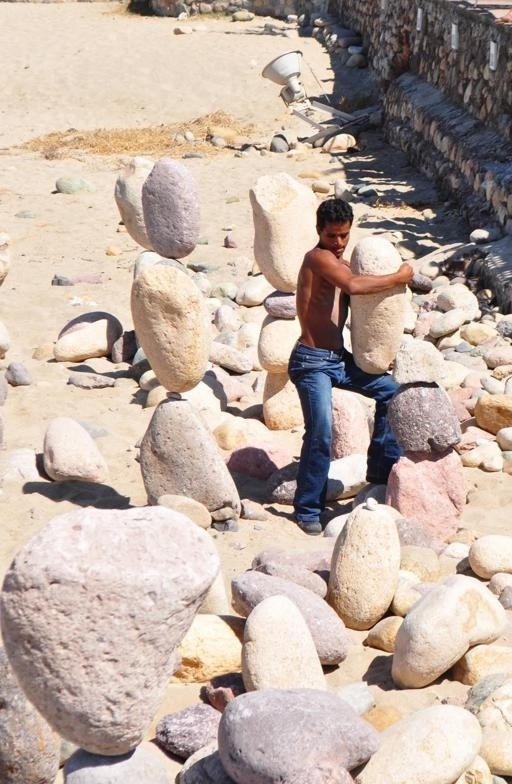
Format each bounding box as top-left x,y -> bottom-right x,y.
295,509 -> 323,535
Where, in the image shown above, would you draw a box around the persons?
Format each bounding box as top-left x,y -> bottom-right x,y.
287,197 -> 414,535
